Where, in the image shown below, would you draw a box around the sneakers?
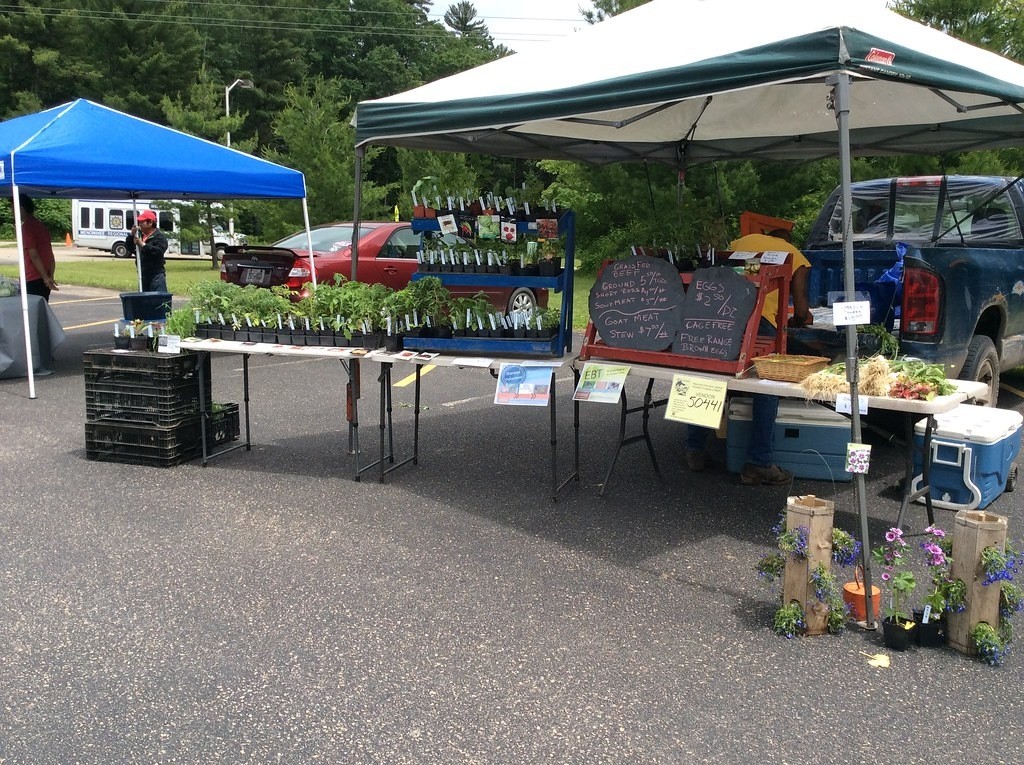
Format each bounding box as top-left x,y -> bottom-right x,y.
686,441 -> 714,471
741,465 -> 794,486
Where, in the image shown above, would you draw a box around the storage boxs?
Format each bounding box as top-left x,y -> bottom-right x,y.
715,391 -> 855,481
82,346 -> 242,468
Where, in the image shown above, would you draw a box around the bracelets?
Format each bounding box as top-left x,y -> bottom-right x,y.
142,242 -> 146,247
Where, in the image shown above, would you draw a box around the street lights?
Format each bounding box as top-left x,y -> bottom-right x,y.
224,78 -> 244,246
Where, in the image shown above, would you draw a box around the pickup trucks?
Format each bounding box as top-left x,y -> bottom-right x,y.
714,173 -> 1024,409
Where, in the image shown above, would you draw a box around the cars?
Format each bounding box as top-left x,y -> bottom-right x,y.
221,220 -> 549,317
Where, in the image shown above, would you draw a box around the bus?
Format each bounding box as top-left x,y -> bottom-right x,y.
72,197 -> 246,259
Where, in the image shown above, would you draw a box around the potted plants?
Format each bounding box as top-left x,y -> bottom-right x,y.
186,273 -> 562,352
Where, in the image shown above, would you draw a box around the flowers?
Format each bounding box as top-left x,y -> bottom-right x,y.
970,536 -> 1024,670
753,506 -> 864,638
870,524 -> 969,625
113,318 -> 166,338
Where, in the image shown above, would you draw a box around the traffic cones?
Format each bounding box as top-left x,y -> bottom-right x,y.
66,232 -> 72,248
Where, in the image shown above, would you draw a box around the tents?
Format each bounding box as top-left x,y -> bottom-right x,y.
347,1 -> 1024,629
0,97 -> 317,398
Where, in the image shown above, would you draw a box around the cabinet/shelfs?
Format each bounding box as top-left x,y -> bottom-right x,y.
578,247 -> 793,381
403,208 -> 574,358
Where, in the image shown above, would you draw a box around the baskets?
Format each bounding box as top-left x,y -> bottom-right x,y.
752,353 -> 831,382
83,346 -> 241,467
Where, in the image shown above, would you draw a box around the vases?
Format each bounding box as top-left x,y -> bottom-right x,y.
882,615 -> 917,652
113,333 -> 159,350
945,511 -> 1009,655
913,610 -> 948,648
783,495 -> 834,637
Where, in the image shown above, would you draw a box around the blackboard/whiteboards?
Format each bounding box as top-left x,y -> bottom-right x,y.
589,255 -> 686,351
672,266 -> 758,362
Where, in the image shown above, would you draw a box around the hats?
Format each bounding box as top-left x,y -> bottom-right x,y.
138,211 -> 156,223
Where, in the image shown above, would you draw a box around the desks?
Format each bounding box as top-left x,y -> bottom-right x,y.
574,369 -> 988,566
0,293 -> 66,380
373,330 -> 585,503
180,336 -> 394,482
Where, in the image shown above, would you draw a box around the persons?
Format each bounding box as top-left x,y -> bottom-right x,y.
126,211 -> 168,292
8,194 -> 59,371
686,228 -> 812,484
